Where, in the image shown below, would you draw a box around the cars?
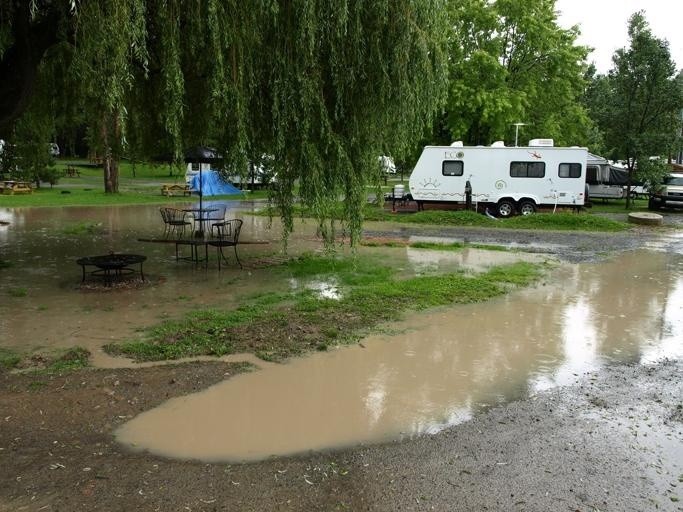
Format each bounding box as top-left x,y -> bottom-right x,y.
650,174 -> 683,210
40,143 -> 60,159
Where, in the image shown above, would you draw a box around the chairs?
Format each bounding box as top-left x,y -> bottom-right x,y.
158,203 -> 243,270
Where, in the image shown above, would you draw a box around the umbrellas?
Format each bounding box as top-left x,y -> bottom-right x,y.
153,144 -> 233,232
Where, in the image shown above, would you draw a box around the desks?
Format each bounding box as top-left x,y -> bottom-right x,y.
78,255 -> 147,286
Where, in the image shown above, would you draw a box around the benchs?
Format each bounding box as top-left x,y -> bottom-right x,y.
161,184 -> 192,198
